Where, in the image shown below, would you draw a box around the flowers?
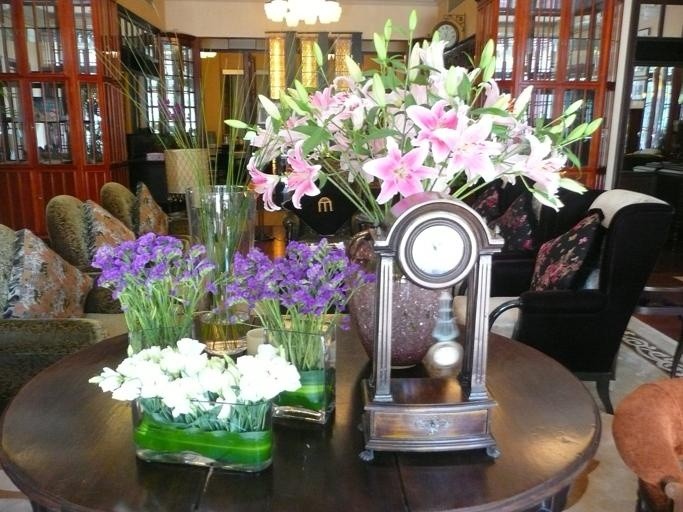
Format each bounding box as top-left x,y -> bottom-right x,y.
222,9 -> 603,230
89,232 -> 378,431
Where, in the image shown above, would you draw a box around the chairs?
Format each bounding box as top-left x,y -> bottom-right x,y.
453,189 -> 672,413
612,378 -> 683,512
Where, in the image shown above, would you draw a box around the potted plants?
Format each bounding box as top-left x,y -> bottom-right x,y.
94,6 -> 341,311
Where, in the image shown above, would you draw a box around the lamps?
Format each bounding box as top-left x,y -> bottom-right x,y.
264,0 -> 342,27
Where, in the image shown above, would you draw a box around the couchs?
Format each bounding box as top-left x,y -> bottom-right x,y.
0,183 -> 187,403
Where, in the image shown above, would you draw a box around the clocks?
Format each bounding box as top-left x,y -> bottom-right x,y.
355,190 -> 506,462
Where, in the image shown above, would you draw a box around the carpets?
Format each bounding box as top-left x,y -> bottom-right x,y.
0,315 -> 683,512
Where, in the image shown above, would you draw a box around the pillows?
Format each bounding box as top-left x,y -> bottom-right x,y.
0,194 -> 122,318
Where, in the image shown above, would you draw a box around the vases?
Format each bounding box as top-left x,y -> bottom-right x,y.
134,417 -> 275,472
344,231 -> 464,370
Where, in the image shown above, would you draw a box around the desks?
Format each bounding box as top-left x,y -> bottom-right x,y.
0,331 -> 601,512
